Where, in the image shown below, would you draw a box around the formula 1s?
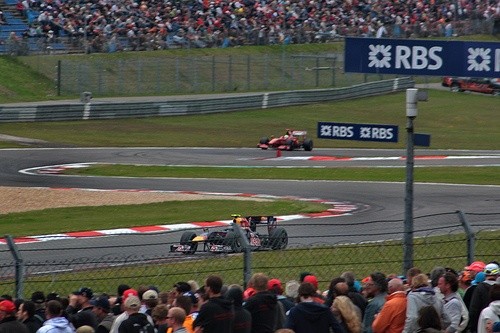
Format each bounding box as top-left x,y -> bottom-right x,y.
255,129 -> 313,151
167,212 -> 288,256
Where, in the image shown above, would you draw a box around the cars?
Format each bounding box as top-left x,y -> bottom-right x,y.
441,76 -> 500,96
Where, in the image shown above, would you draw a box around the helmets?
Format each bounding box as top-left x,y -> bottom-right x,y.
233,218 -> 249,227
285,129 -> 291,135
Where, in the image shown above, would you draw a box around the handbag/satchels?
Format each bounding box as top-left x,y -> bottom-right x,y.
418,305 -> 442,331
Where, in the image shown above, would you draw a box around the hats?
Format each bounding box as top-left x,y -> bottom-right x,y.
121,289 -> 142,309
445,259 -> 500,284
73,288 -> 92,298
47,293 -> 60,301
142,290 -> 158,300
0,300 -> 16,314
76,311 -> 97,328
31,290 -> 45,303
89,295 -> 110,310
267,272 -> 318,298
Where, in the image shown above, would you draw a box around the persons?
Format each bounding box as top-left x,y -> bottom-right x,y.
0,0 -> 500,53
233,217 -> 252,234
286,129 -> 293,137
0,261 -> 500,333
